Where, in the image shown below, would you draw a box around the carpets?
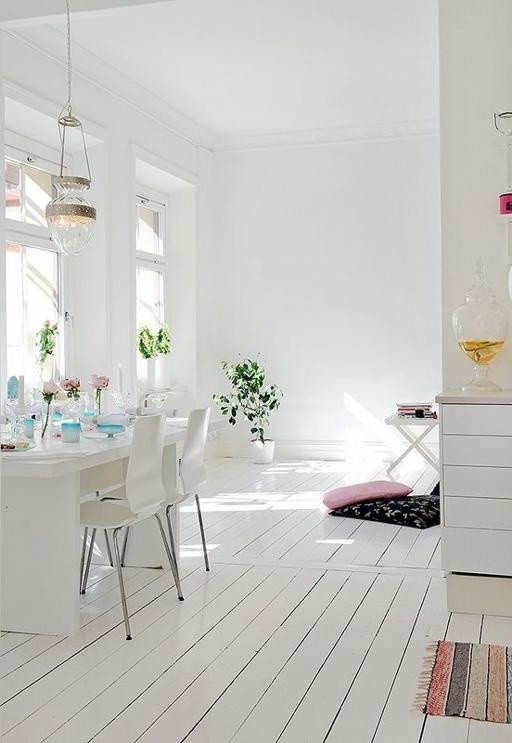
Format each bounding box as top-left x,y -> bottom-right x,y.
416,641 -> 511,725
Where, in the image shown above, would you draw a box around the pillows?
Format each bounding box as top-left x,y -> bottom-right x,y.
321,480 -> 439,528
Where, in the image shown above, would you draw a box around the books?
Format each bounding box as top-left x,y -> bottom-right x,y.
394,400 -> 435,418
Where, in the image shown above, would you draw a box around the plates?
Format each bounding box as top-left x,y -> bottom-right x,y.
0,439 -> 38,452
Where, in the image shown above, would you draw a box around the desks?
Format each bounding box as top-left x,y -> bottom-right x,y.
383,413 -> 441,475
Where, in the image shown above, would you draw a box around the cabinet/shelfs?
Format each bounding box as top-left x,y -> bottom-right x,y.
433,388 -> 512,618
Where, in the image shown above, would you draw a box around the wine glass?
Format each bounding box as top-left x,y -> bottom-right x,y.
95,422 -> 124,442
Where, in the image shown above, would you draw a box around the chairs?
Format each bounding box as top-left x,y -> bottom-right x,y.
81,405 -> 214,642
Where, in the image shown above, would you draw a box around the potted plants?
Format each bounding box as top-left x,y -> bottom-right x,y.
210,352 -> 285,464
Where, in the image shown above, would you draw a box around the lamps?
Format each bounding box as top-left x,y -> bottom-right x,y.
45,3 -> 99,263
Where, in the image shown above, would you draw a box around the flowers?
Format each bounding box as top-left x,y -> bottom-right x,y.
39,374 -> 110,439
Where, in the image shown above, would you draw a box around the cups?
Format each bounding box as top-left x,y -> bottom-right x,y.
60,422 -> 81,444
23,419 -> 34,439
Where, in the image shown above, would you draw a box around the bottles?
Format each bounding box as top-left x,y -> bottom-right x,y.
450,258 -> 508,397
